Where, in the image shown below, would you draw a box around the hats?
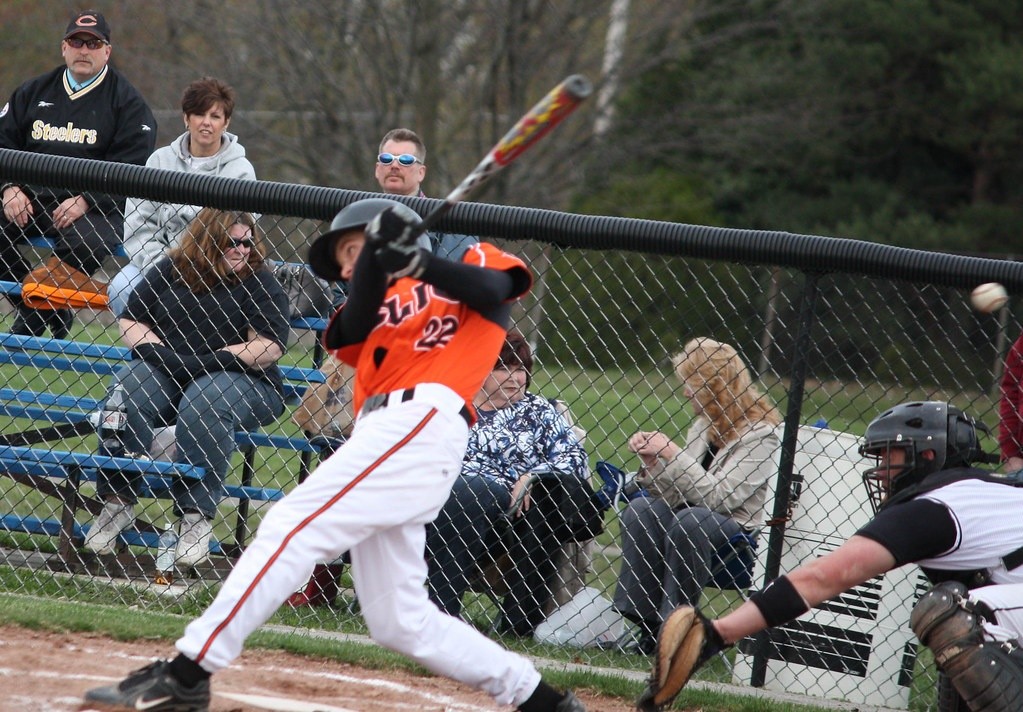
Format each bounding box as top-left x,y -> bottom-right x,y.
64,9 -> 110,43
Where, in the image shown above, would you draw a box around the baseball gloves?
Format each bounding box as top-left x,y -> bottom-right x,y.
633,602 -> 736,712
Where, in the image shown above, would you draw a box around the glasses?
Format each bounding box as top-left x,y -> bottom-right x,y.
227,237 -> 254,248
378,153 -> 423,167
65,37 -> 103,50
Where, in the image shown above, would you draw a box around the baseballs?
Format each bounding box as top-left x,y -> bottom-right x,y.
970,282 -> 1009,314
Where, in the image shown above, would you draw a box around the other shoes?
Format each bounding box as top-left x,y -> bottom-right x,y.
623,631 -> 659,657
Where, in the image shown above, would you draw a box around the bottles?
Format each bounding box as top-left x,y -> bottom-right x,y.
156,524 -> 177,584
100,384 -> 127,458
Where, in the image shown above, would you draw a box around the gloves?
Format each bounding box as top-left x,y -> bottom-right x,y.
363,207 -> 432,281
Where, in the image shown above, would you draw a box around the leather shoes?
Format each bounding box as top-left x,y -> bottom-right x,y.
10,305 -> 73,340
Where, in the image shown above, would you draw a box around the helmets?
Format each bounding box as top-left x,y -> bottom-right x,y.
857,401 -> 979,518
308,198 -> 432,283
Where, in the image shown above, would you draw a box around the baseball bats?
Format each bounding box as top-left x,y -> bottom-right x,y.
402,70 -> 595,248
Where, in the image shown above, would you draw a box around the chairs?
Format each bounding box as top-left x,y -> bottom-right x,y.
596,419 -> 829,671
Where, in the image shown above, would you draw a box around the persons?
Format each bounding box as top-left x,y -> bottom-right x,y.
634,401 -> 1023,712
610,337 -> 783,659
425,322 -> 604,636
283,129 -> 479,607
78,207 -> 289,566
83,199 -> 584,712
107,77 -> 264,322
0,10 -> 158,349
1000,329 -> 1023,474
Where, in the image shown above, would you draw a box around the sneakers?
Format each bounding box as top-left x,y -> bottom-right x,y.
84,502 -> 136,556
553,689 -> 586,712
176,513 -> 213,566
84,657 -> 210,712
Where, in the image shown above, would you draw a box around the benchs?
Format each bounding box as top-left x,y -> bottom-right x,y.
0,236 -> 338,570
